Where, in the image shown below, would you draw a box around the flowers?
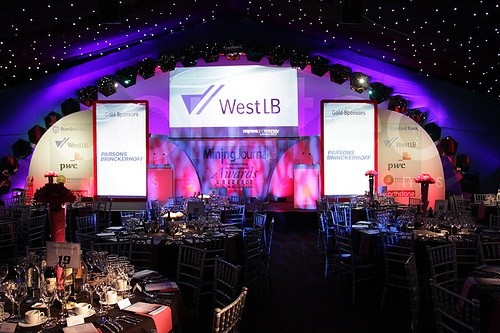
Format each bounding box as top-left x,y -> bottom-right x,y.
366,170 -> 377,176
35,182 -> 76,207
414,174 -> 437,185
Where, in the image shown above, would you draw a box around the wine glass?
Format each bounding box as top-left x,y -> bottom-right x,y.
82,250 -> 135,316
40,281 -> 71,329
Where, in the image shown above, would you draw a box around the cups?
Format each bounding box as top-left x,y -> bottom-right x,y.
104,290 -> 118,303
75,302 -> 91,315
378,211 -> 473,234
116,279 -> 127,290
25,310 -> 45,324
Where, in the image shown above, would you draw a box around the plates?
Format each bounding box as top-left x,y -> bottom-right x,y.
68,307 -> 95,318
18,315 -> 48,327
111,285 -> 132,291
99,296 -> 123,305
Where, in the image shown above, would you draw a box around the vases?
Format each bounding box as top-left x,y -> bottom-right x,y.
51,204 -> 62,211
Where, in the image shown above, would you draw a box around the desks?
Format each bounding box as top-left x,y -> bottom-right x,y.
0,251 -> 186,333
466,265 -> 500,333
352,217 -> 490,292
99,221 -> 256,274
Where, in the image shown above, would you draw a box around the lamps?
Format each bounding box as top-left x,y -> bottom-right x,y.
0,38 -> 245,195
246,40 -> 471,175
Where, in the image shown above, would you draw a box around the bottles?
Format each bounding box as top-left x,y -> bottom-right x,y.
25,252 -> 74,297
0,257 -> 29,323
413,205 -> 422,230
166,211 -> 172,224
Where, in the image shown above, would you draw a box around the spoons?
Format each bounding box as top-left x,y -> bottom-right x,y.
96,316 -> 123,333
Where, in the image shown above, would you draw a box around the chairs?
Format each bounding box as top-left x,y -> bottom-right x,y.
315,194 -> 500,333
0,186 -> 275,333
